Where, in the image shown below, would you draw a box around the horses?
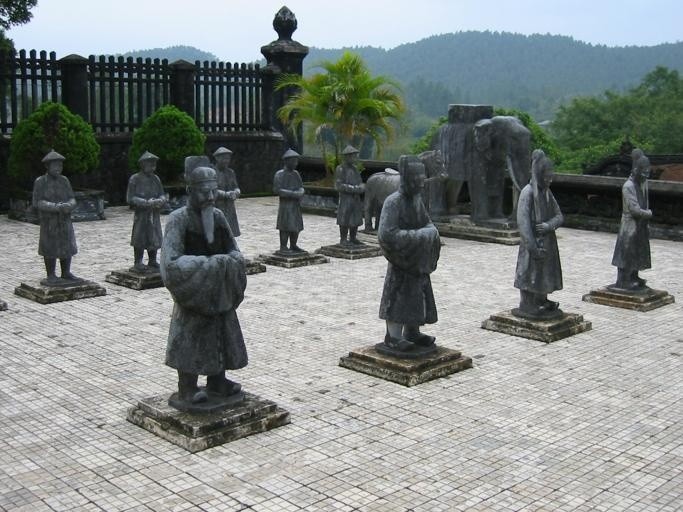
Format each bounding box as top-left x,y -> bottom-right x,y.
364,150 -> 450,233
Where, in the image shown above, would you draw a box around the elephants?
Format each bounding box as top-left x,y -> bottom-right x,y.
429,116 -> 532,223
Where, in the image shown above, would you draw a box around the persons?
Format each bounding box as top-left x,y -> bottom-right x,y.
32,149 -> 78,283
272,148 -> 304,252
212,146 -> 242,236
159,156 -> 247,403
335,145 -> 366,246
378,155 -> 441,351
126,150 -> 166,270
611,148 -> 653,290
513,149 -> 564,316
